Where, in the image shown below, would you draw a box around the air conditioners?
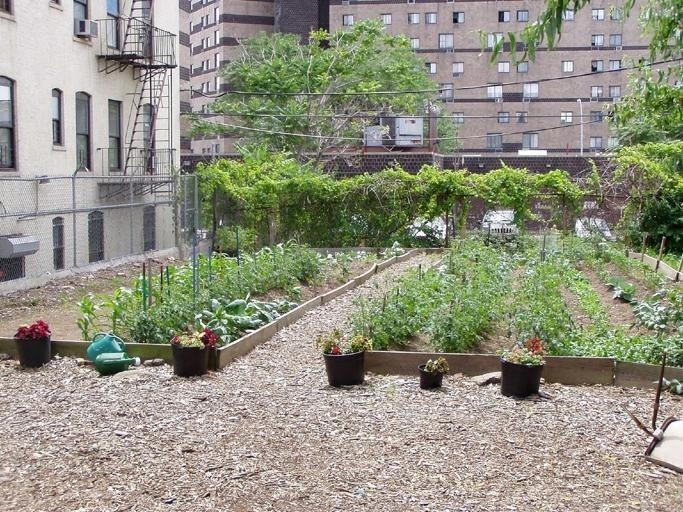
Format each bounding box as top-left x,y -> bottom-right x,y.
74,19 -> 98,39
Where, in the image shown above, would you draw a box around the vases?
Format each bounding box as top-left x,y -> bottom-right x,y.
500,361 -> 545,397
322,352 -> 365,386
174,350 -> 205,376
15,340 -> 50,367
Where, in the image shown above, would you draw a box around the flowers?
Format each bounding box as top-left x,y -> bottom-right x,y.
168,330 -> 216,352
502,338 -> 547,367
315,327 -> 373,356
12,319 -> 51,340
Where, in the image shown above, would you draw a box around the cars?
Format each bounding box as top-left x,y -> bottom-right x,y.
576,217 -> 611,238
410,214 -> 447,247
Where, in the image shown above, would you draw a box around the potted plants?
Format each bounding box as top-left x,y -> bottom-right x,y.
416,355 -> 450,389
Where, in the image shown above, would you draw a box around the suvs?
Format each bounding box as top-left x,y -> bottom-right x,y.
476,208 -> 528,240
